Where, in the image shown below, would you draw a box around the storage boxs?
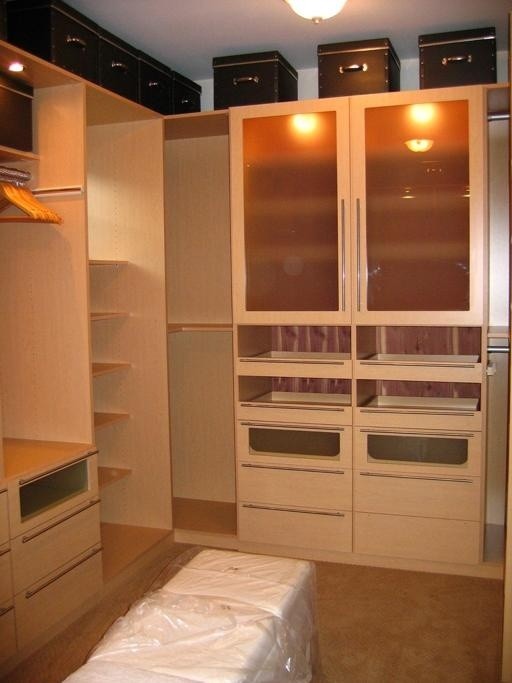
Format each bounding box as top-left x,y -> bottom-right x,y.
4,0 -> 201,113
210,24 -> 497,109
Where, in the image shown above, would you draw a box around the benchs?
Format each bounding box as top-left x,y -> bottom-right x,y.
61,547 -> 327,683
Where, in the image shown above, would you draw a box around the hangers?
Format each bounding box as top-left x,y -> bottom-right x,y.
0,166 -> 65,225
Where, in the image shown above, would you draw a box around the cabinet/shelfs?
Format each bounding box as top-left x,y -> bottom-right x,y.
160,83 -> 511,582
0,39 -> 177,681
